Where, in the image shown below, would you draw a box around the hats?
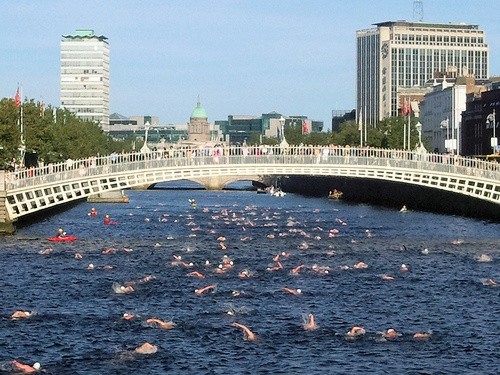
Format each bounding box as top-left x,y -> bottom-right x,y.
63,232 -> 66,234
106,215 -> 109,218
59,229 -> 64,232
91,208 -> 95,210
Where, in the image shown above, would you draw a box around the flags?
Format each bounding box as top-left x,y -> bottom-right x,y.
401,102 -> 411,115
14,88 -> 20,107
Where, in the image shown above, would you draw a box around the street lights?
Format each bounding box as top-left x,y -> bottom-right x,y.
139,121 -> 151,153
439,116 -> 449,154
278,116 -> 289,152
413,121 -> 427,155
486,109 -> 496,155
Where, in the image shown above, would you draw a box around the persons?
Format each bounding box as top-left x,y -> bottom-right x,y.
229,141 -> 280,156
110,146 -> 158,163
14,360 -> 40,373
8,158 -> 44,178
377,146 -> 413,160
202,143 -> 224,156
162,145 -> 199,158
11,198 -> 432,354
64,152 -> 101,170
427,152 -> 500,172
290,143 -> 376,157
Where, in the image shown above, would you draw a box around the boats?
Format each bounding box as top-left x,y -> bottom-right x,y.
104,221 -> 116,225
191,203 -> 197,207
400,208 -> 409,214
47,235 -> 77,242
87,211 -> 98,216
256,185 -> 286,197
327,189 -> 343,199
